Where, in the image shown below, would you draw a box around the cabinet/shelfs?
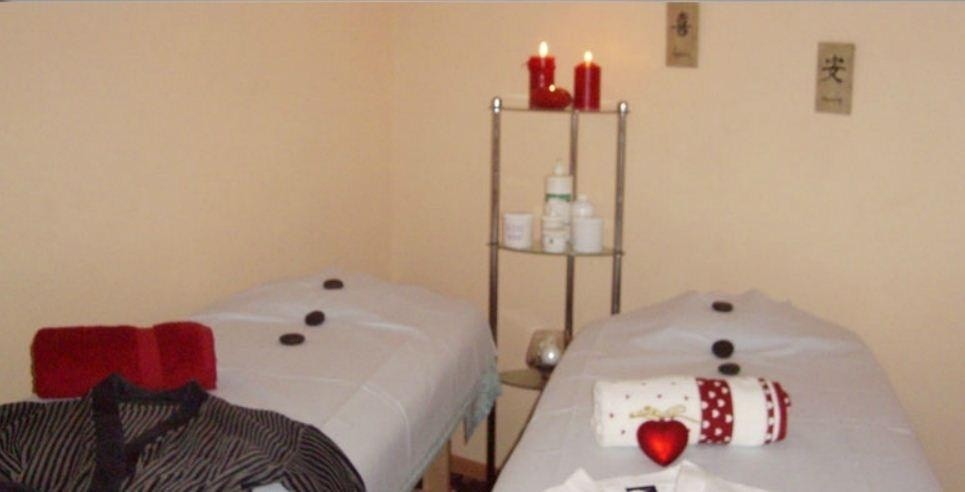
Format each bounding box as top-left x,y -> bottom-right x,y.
484,99 -> 625,491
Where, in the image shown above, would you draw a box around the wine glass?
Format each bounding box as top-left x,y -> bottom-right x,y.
526,329 -> 576,384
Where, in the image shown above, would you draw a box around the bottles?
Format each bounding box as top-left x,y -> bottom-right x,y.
502,157 -> 603,254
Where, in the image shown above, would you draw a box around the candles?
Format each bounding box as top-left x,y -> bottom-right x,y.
526,39 -> 604,113
